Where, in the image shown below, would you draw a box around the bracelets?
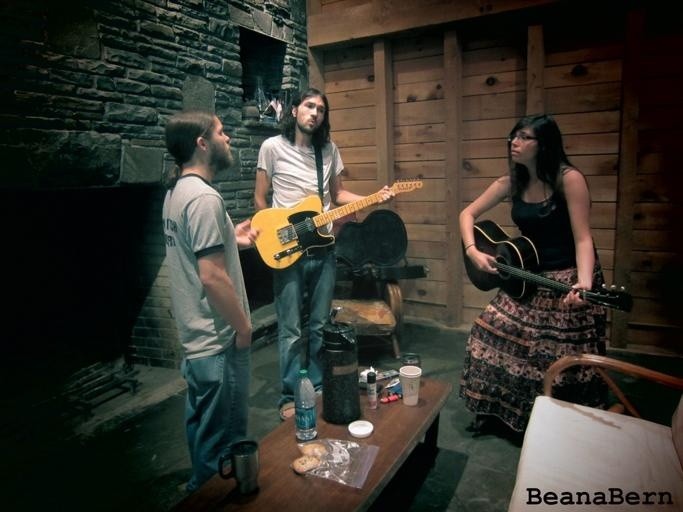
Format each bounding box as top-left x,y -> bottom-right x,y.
465,243 -> 473,249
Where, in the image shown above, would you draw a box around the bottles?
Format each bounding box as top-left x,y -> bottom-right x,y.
321,319 -> 360,424
366,372 -> 377,410
294,369 -> 317,440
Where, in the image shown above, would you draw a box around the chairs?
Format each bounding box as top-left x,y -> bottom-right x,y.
506,351 -> 681,512
329,280 -> 405,361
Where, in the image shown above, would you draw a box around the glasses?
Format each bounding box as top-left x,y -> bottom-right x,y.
505,132 -> 539,142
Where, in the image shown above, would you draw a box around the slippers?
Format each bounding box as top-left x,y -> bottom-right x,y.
280,402 -> 298,421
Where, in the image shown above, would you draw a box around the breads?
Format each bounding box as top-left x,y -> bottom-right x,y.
300,444 -> 328,457
292,456 -> 319,474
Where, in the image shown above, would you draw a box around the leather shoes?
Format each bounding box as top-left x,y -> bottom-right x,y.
464,415 -> 504,434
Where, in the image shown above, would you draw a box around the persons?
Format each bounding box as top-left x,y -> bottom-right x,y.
255,87 -> 394,420
459,112 -> 607,436
162,111 -> 260,494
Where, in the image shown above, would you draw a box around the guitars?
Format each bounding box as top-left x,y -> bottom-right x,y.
250,176 -> 423,270
461,218 -> 627,311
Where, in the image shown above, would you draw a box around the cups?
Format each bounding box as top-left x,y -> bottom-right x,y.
399,365 -> 422,406
218,440 -> 260,492
